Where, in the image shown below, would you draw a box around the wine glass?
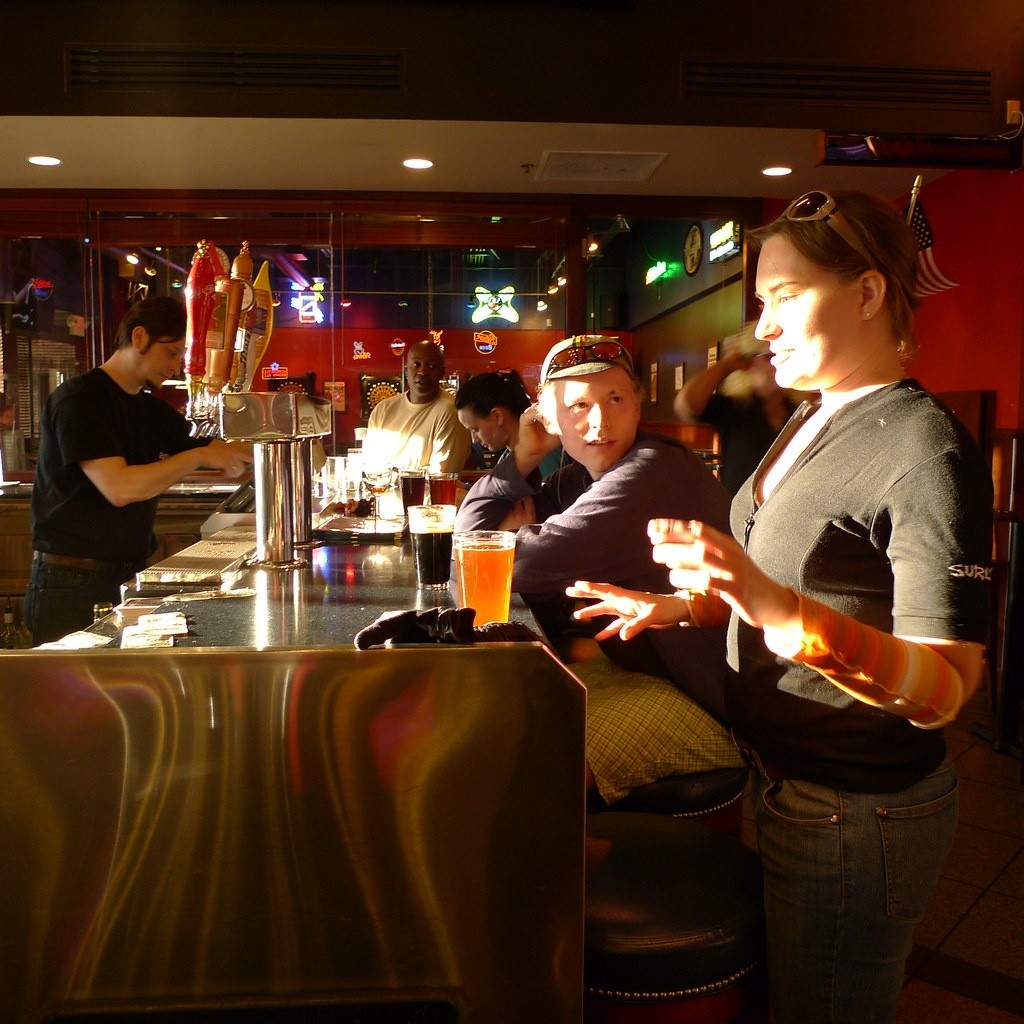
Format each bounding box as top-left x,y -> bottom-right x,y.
363,469 -> 392,519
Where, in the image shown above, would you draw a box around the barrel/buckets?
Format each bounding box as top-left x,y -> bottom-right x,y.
255,442 -> 311,564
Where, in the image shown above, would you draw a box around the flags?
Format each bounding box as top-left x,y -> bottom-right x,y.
900,196 -> 963,301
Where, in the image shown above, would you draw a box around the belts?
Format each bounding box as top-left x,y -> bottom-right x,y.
32,549 -> 134,574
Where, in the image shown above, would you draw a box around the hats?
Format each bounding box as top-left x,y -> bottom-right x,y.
540,335 -> 635,387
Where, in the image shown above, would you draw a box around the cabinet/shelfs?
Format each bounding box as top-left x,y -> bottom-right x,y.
0,500 -> 222,597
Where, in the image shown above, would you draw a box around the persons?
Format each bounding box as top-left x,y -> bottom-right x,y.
564,186 -> 998,1023
364,340 -> 472,483
453,332 -> 729,808
20,294 -> 254,650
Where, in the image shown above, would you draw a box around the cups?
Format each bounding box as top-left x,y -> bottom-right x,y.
429,472 -> 458,504
407,505 -> 457,587
401,471 -> 425,517
453,530 -> 516,629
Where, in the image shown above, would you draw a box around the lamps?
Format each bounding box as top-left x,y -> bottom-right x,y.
535,231 -> 599,311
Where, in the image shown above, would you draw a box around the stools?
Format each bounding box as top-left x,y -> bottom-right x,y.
586,768 -> 769,1004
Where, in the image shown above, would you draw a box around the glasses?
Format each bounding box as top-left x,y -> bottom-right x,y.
545,341 -> 635,378
779,191 -> 875,271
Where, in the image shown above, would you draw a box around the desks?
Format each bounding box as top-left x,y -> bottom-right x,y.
0,525 -> 586,1024
